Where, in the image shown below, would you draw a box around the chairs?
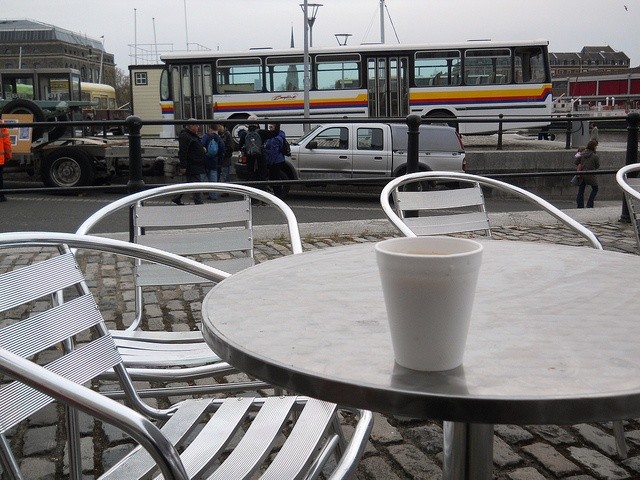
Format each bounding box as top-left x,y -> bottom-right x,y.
378,170 -> 604,248
613,163 -> 640,253
0,229 -> 375,479
50,180 -> 302,479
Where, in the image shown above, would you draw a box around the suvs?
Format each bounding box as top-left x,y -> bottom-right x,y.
234,123 -> 467,198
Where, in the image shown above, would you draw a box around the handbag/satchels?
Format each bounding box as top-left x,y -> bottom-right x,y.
282,137 -> 291,157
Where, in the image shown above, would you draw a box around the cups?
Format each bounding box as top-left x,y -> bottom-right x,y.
374,234 -> 484,373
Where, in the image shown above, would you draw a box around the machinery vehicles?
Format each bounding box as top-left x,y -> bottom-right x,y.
0,68 -> 159,196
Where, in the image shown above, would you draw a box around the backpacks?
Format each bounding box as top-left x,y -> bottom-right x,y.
206,132 -> 220,158
245,129 -> 263,158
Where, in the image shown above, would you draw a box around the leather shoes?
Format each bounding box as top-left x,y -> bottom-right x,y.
172,197 -> 185,204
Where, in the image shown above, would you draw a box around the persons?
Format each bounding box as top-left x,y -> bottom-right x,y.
237,115 -> 265,181
575,144 -> 583,177
590,123 -> 599,144
215,120 -> 233,197
262,123 -> 290,198
201,122 -> 224,200
571,141 -> 598,208
0,113 -> 13,201
172,118 -> 205,205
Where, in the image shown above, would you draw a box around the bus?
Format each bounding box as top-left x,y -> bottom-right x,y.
50,80 -> 123,136
160,40 -> 553,150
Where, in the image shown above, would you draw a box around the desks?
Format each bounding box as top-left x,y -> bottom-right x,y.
200,236 -> 640,480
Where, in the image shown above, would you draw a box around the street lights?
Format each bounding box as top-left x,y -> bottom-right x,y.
334,34 -> 352,88
300,3 -> 322,47
